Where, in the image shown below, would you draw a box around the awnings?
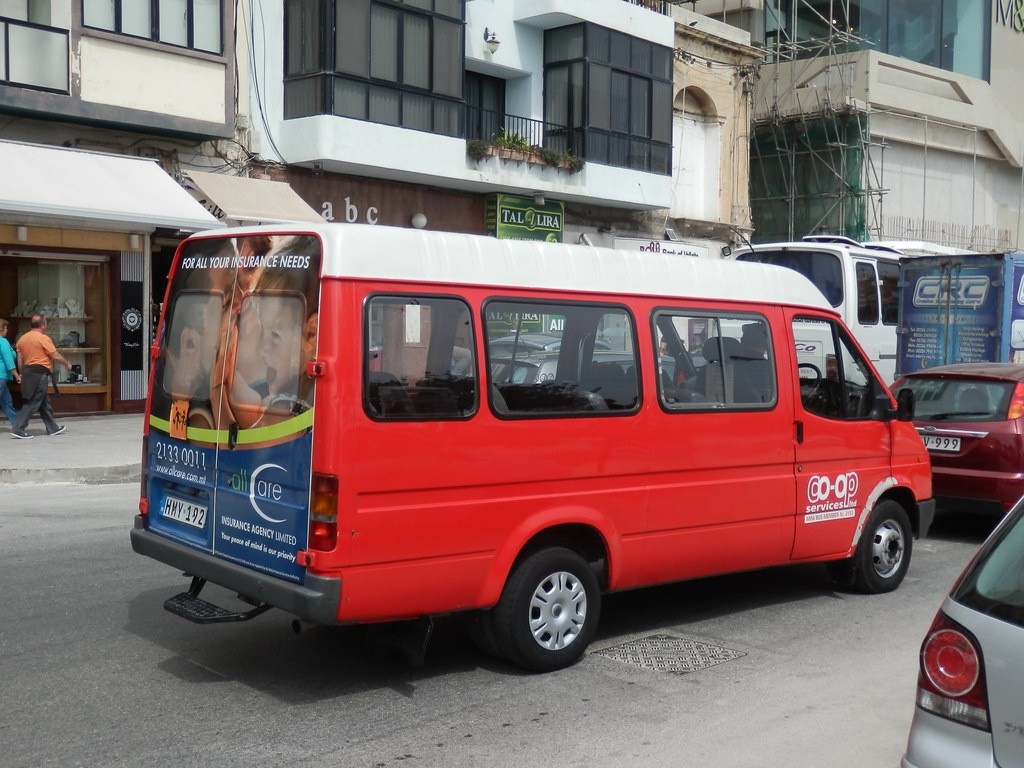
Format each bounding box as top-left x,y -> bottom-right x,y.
0,137 -> 227,229
182,162 -> 326,222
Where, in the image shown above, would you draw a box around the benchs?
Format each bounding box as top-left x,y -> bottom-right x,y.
369,359 -> 703,420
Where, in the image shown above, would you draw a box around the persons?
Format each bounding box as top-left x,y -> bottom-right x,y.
0,319 -> 21,423
213,235 -> 322,411
11,316 -> 72,437
732,322 -> 814,399
158,235 -> 273,404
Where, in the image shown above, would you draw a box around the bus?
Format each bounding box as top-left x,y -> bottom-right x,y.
729,234 -> 983,393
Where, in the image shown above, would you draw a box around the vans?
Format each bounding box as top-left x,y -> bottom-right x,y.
131,224 -> 937,675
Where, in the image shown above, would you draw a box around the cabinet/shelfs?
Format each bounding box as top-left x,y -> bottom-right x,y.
6,311 -> 106,397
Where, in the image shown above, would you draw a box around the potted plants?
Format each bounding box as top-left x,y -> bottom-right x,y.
468,126 -> 587,173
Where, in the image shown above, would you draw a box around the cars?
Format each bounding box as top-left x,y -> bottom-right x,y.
898,496 -> 1024,768
886,363 -> 1023,530
452,333 -> 675,383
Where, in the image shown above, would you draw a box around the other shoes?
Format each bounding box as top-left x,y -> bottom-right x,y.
49,425 -> 66,435
10,430 -> 34,439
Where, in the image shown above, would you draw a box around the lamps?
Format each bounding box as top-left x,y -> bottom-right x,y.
484,27 -> 500,54
664,228 -> 677,242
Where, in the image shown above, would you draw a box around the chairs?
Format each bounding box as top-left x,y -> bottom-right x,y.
957,387 -> 991,415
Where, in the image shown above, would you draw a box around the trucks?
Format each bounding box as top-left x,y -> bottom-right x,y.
894,252 -> 1024,380
